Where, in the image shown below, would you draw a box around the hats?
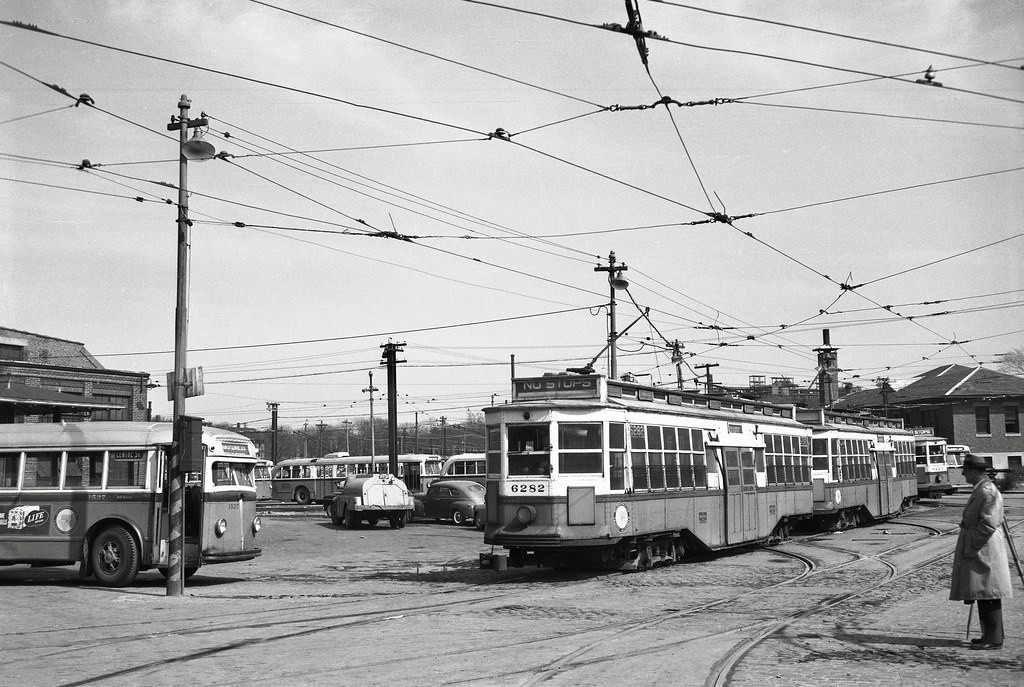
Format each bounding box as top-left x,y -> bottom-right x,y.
957,454 -> 991,470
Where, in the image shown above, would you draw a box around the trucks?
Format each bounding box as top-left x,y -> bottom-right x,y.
328,473 -> 416,531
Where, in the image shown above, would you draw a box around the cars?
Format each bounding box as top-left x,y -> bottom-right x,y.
415,480 -> 486,527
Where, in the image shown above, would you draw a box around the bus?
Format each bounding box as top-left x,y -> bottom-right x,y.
480,363 -> 919,575
437,451 -> 487,487
218,459 -> 274,501
272,451 -> 446,506
0,418 -> 260,589
906,425 -> 975,501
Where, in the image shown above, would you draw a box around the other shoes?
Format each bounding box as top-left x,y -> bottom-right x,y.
970,642 -> 1003,650
970,638 -> 983,644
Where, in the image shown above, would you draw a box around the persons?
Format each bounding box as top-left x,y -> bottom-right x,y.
948,453 -> 1013,649
